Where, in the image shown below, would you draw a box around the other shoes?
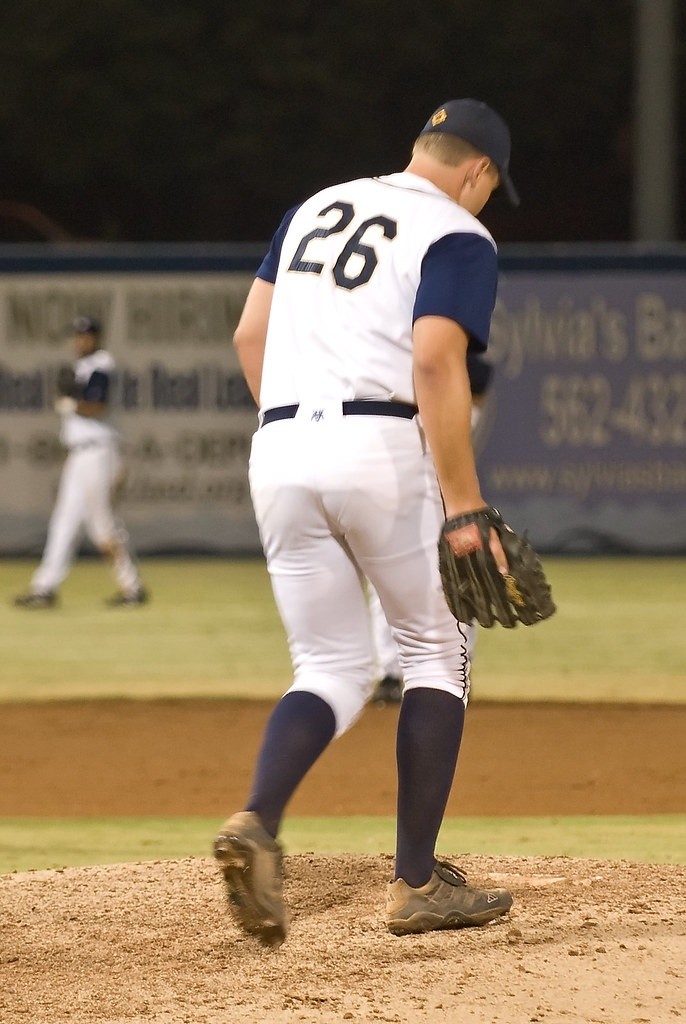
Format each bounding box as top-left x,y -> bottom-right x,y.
16,591 -> 57,611
102,589 -> 154,606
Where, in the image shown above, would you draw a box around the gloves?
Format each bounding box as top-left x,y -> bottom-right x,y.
52,397 -> 78,417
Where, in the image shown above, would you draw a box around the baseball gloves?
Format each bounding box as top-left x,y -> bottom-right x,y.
439,506 -> 557,629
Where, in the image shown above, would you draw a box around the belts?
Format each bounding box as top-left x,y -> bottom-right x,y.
261,400 -> 419,428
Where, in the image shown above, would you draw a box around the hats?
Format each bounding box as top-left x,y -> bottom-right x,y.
70,315 -> 103,335
417,97 -> 521,207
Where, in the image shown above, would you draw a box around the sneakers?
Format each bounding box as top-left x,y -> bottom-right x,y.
213,811 -> 287,946
385,857 -> 513,937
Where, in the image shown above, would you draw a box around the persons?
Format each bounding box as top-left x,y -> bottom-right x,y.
214,101 -> 556,948
13,315 -> 147,609
366,341 -> 491,704
